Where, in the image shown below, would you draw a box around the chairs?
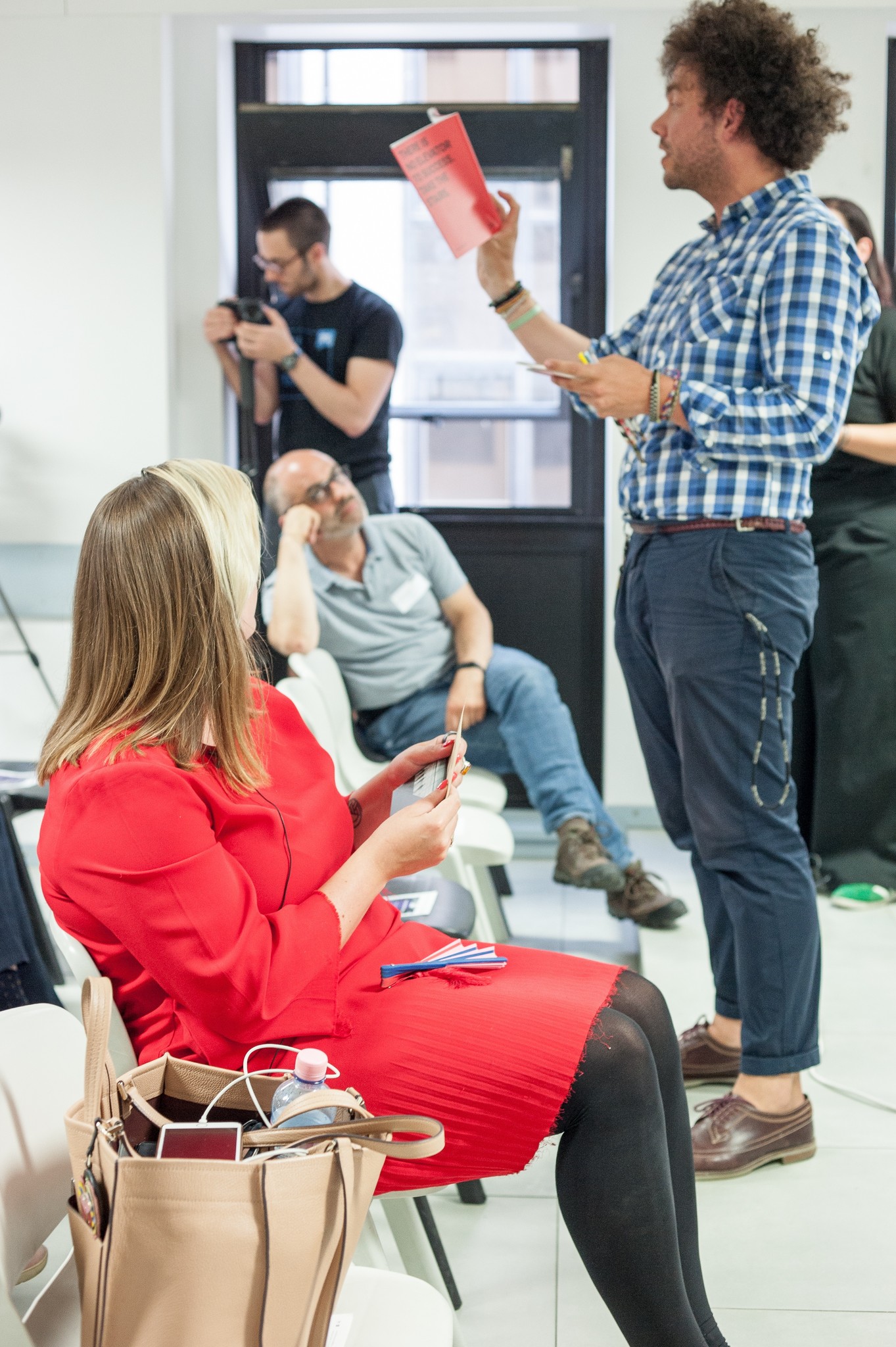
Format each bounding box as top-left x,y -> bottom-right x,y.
0,650 -> 516,1347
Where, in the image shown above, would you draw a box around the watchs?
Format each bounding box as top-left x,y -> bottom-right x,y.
279,346 -> 304,371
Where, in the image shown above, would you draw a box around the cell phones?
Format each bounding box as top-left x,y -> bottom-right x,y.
155,1122 -> 244,1161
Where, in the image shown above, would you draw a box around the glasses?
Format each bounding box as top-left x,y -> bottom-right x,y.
283,462 -> 352,516
252,243 -> 318,274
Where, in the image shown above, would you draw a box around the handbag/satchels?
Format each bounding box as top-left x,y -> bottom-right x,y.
62,977 -> 446,1347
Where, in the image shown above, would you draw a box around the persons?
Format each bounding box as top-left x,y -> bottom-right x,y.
203,197 -> 402,685
36,457 -> 731,1347
792,192 -> 896,913
259,448 -> 691,926
467,0 -> 880,1185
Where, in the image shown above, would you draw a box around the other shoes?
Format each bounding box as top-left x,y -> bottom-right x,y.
677,1014 -> 786,1089
604,862 -> 690,925
831,880 -> 896,911
552,833 -> 624,894
684,1091 -> 817,1179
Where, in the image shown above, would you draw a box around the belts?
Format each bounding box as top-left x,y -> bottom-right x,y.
625,514 -> 809,535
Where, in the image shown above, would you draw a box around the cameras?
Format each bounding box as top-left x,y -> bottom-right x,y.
217,298 -> 267,324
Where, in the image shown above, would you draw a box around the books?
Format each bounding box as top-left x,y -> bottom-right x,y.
389,107 -> 504,258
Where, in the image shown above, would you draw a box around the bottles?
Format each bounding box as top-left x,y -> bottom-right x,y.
269,1047 -> 337,1160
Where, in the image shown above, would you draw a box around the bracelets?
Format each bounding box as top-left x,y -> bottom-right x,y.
488,280 -> 545,332
647,364 -> 683,420
451,661 -> 487,677
